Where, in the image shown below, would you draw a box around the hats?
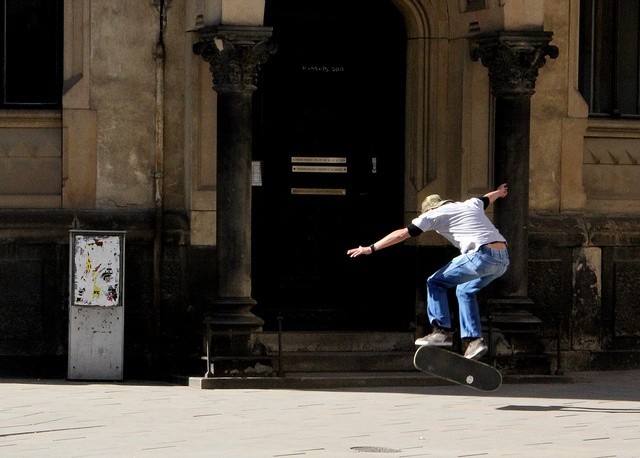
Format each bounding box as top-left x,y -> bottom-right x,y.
421,194 -> 454,214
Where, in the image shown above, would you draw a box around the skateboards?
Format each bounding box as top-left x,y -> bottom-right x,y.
413,346 -> 502,392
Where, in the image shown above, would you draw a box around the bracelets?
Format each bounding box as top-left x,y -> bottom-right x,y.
369,244 -> 376,254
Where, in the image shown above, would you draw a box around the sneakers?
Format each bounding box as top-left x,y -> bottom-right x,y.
464,338 -> 488,361
415,332 -> 454,346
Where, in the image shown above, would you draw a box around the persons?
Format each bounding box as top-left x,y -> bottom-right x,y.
347,184 -> 510,361
